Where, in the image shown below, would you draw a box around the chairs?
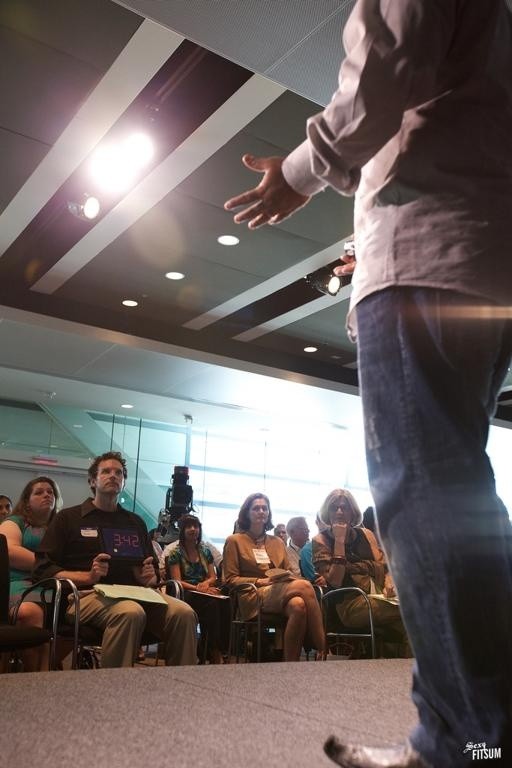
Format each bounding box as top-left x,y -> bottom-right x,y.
40,576 -> 181,669
322,588 -> 404,660
228,583 -> 323,662
177,580 -> 212,664
0,533 -> 62,674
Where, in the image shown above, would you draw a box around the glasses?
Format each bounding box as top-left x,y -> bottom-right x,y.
329,504 -> 350,512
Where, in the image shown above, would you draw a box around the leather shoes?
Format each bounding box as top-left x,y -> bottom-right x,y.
324,734 -> 435,768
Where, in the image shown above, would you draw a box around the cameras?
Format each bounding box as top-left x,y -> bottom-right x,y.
165,464 -> 194,525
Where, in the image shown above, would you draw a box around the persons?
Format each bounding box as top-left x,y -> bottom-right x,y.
300,511 -> 328,599
149,506 -> 380,581
32,452 -> 200,667
284,517 -> 310,576
0,495 -> 13,524
225,1 -> 512,768
0,476 -> 63,673
224,493 -> 332,661
168,515 -> 225,664
312,490 -> 413,659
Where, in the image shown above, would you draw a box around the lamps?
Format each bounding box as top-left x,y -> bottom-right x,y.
67,191 -> 102,223
304,272 -> 342,296
